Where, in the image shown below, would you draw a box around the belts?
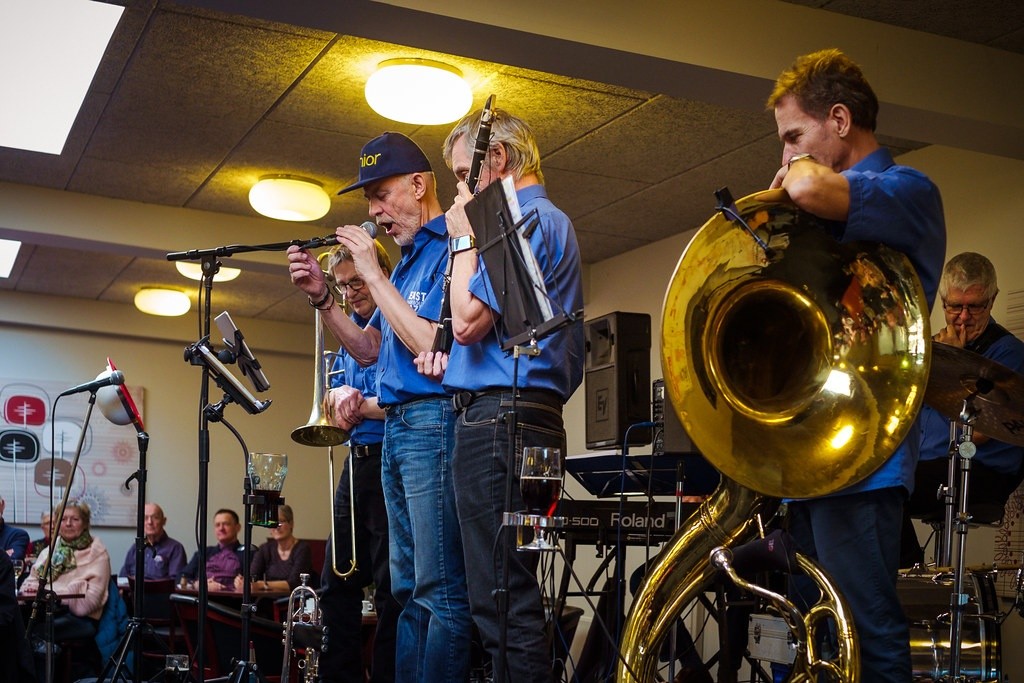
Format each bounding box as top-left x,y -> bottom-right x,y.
451,388 -> 563,414
351,442 -> 382,457
385,393 -> 453,417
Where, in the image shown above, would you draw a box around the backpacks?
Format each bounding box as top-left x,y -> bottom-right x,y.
31,608 -> 97,644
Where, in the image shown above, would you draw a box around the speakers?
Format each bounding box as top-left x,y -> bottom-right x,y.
583,311 -> 653,450
652,378 -> 700,454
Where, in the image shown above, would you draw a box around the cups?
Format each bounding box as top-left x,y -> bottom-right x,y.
362,600 -> 373,611
247,453 -> 289,529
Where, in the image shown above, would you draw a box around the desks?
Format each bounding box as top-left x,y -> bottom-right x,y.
363,612 -> 379,683
18,590 -> 84,600
174,586 -> 284,600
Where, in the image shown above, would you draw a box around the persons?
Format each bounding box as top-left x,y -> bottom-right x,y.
118,501 -> 187,617
319,238 -> 403,683
0,497 -> 29,569
17,499 -> 111,647
25,511 -> 56,561
179,509 -> 259,682
0,548 -> 31,682
899,251 -> 1024,564
234,504 -> 313,677
413,109 -> 585,683
766,46 -> 946,683
288,132 -> 471,683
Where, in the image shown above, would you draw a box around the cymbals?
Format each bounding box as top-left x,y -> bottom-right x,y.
923,341 -> 1024,447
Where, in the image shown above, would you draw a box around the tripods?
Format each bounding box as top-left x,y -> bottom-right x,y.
98,390 -> 199,683
204,400 -> 269,683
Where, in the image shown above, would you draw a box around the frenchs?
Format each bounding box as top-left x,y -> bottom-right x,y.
616,189 -> 933,683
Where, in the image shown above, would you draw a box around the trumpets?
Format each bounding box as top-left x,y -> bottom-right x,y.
280,573 -> 329,683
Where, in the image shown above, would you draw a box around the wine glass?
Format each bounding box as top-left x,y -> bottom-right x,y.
517,446 -> 565,552
11,553 -> 36,596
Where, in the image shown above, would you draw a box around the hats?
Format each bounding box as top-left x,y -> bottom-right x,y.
337,132 -> 435,195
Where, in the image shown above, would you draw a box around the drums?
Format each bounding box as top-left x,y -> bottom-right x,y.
894,563 -> 1003,683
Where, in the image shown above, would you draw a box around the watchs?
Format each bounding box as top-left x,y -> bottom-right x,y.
787,152 -> 817,170
450,234 -> 479,255
263,581 -> 268,590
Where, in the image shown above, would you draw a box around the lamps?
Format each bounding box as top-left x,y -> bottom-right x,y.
248,174 -> 332,221
364,57 -> 473,126
134,287 -> 192,316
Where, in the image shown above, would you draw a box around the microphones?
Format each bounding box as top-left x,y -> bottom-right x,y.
304,222 -> 377,249
60,369 -> 125,396
713,186 -> 738,221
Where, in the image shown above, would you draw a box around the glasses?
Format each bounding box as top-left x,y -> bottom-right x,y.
943,297 -> 991,314
333,277 -> 364,295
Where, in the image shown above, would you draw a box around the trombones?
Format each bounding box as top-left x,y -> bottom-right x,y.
290,252 -> 358,578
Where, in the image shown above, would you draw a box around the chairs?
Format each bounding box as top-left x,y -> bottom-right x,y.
109,535 -> 329,683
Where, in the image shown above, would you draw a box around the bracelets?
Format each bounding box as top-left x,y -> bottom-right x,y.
309,282 -> 334,310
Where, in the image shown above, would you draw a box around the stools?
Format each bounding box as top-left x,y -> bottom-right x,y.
907,502 -> 1006,683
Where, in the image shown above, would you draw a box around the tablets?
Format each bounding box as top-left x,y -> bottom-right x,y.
214,311 -> 270,391
202,346 -> 263,410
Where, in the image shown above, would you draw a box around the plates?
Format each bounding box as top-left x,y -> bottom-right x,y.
362,611 -> 377,617
